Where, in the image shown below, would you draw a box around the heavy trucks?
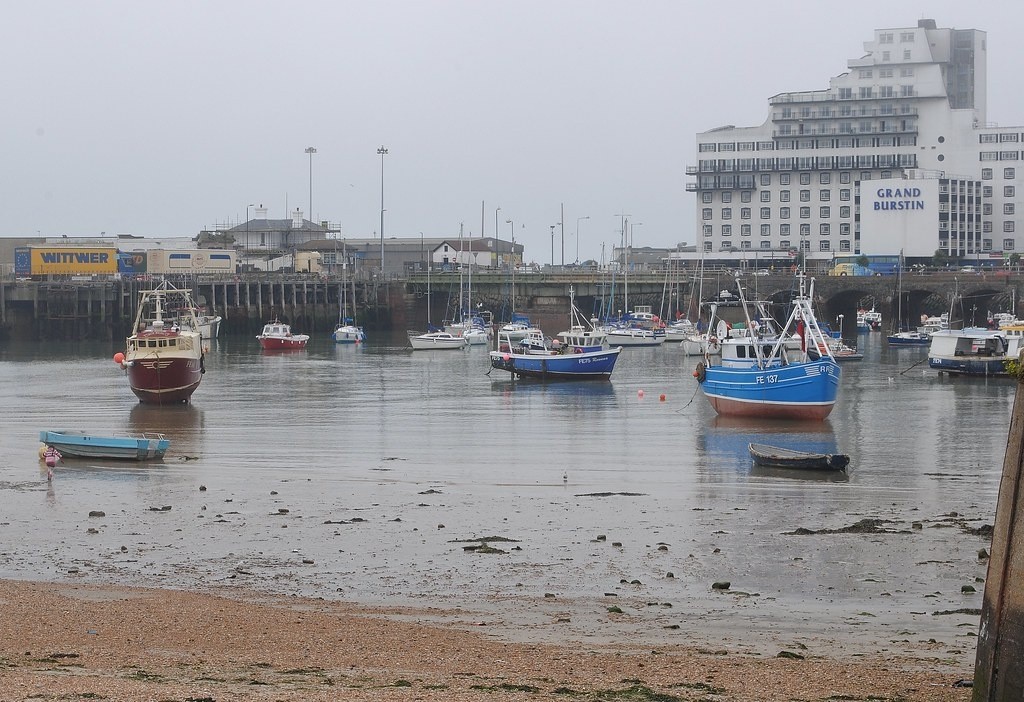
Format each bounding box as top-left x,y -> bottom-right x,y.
144,248 -> 247,280
13,246 -> 135,283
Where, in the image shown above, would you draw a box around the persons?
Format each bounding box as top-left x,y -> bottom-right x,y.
43,446 -> 65,481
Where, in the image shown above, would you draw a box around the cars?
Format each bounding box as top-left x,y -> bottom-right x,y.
751,268 -> 772,276
960,264 -> 976,275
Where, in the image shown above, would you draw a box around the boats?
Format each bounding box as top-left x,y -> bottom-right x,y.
179,314 -> 222,339
404,223 -> 884,384
886,275 -> 1024,380
487,281 -> 624,383
692,276 -> 842,422
36,430 -> 170,463
112,278 -> 206,405
255,313 -> 310,350
747,440 -> 851,472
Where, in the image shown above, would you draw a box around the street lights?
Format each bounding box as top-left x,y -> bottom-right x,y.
246,204 -> 254,271
495,207 -> 502,268
613,214 -> 631,272
631,223 -> 643,271
550,225 -> 556,265
506,220 -> 514,274
576,216 -> 590,265
380,209 -> 388,271
305,146 -> 318,241
376,144 -> 388,270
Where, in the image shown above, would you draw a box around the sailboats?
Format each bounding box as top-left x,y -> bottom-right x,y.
330,259 -> 368,344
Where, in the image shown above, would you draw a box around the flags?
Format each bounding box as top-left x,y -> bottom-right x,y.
794,320 -> 807,353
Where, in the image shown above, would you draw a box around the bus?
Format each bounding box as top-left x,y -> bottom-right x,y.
827,255 -> 900,277
